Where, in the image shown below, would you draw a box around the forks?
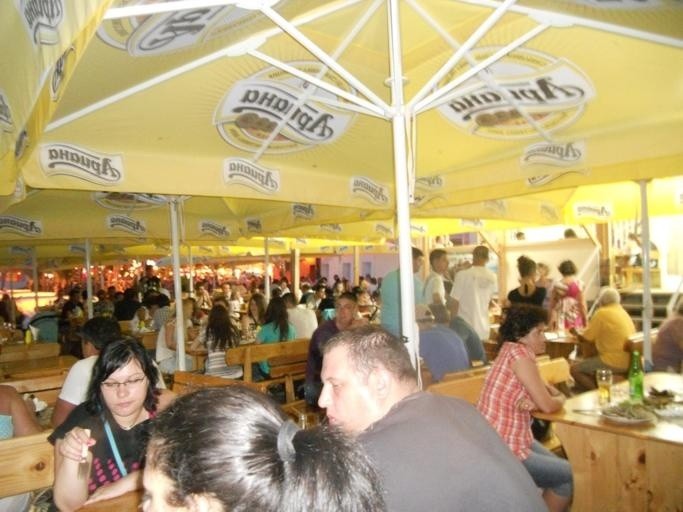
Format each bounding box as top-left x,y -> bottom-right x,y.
78,429 -> 91,480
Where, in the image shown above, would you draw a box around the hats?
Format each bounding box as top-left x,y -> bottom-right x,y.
416,304 -> 435,320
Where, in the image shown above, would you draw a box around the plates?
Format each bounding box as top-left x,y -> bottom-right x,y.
599,405 -> 654,424
642,391 -> 683,418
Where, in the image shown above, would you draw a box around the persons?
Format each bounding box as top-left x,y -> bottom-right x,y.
477,304 -> 573,512
2,229 -> 682,425
44,333 -> 158,512
137,381 -> 389,512
0,383 -> 46,512
314,324 -> 550,512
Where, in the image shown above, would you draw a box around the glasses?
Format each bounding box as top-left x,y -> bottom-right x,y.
99,374 -> 145,390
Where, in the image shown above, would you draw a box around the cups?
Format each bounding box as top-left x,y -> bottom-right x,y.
596,370 -> 613,405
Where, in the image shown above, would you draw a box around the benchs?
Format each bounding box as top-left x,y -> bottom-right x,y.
0,327 -> 144,512
481,326 -> 660,371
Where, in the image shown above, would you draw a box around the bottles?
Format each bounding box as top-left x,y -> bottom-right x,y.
297,414 -> 307,430
629,351 -> 643,401
247,323 -> 256,341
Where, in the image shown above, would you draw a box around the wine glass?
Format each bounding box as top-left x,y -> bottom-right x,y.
4,322 -> 18,345
